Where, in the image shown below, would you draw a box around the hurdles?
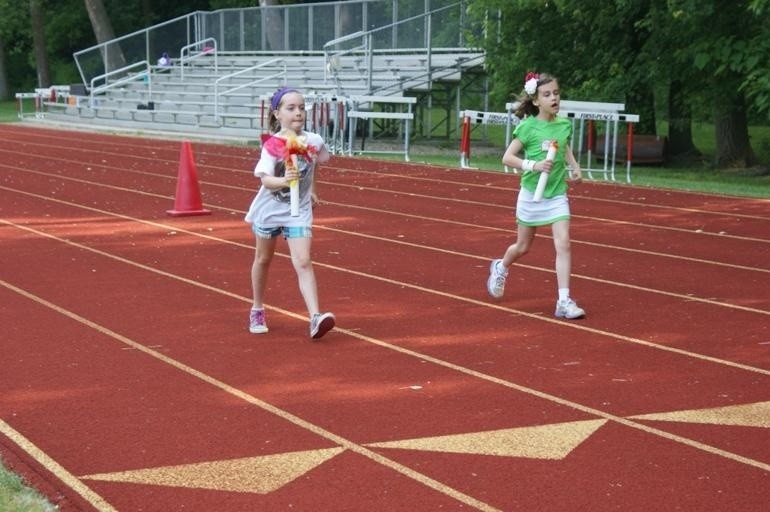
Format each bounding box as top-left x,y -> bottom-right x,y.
458,100 -> 640,185
16,86 -> 70,119
259,92 -> 417,161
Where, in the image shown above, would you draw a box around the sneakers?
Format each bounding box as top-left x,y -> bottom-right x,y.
554,298 -> 586,320
487,259 -> 508,299
310,312 -> 336,339
249,307 -> 269,334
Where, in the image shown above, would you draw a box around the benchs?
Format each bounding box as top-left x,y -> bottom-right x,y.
65,53 -> 486,131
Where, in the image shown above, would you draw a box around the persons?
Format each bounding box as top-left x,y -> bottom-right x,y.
244,87 -> 336,339
157,52 -> 171,74
204,41 -> 215,56
485,71 -> 587,319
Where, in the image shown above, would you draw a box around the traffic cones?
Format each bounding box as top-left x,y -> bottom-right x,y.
166,141 -> 212,217
